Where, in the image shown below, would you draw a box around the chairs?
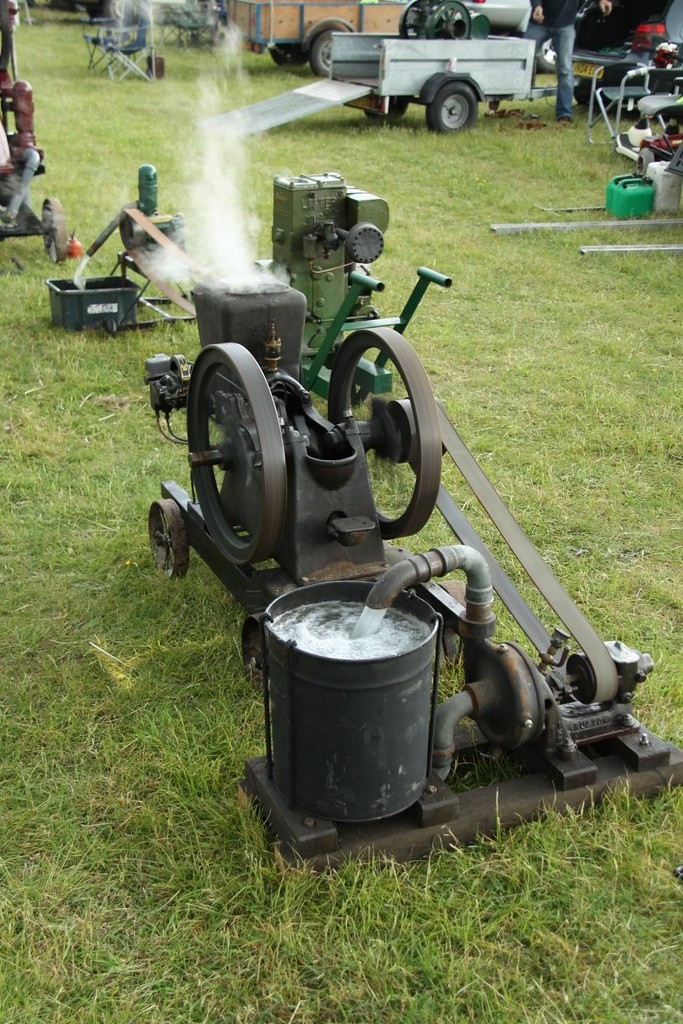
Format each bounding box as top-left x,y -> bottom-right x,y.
79,4 -> 216,83
586,35 -> 683,147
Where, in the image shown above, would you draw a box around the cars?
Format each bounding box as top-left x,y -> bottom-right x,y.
572,0 -> 683,104
433,0 -> 560,73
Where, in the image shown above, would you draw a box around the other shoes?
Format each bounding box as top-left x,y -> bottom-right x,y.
558,115 -> 574,123
489,101 -> 499,110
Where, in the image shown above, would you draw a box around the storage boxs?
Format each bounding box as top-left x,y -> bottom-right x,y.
45,276 -> 142,333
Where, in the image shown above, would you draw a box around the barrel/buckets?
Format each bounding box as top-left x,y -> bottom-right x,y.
265,579 -> 439,825
611,178 -> 655,217
606,172 -> 643,211
646,162 -> 678,215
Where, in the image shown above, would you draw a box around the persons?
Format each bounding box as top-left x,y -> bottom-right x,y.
489,0 -> 613,126
